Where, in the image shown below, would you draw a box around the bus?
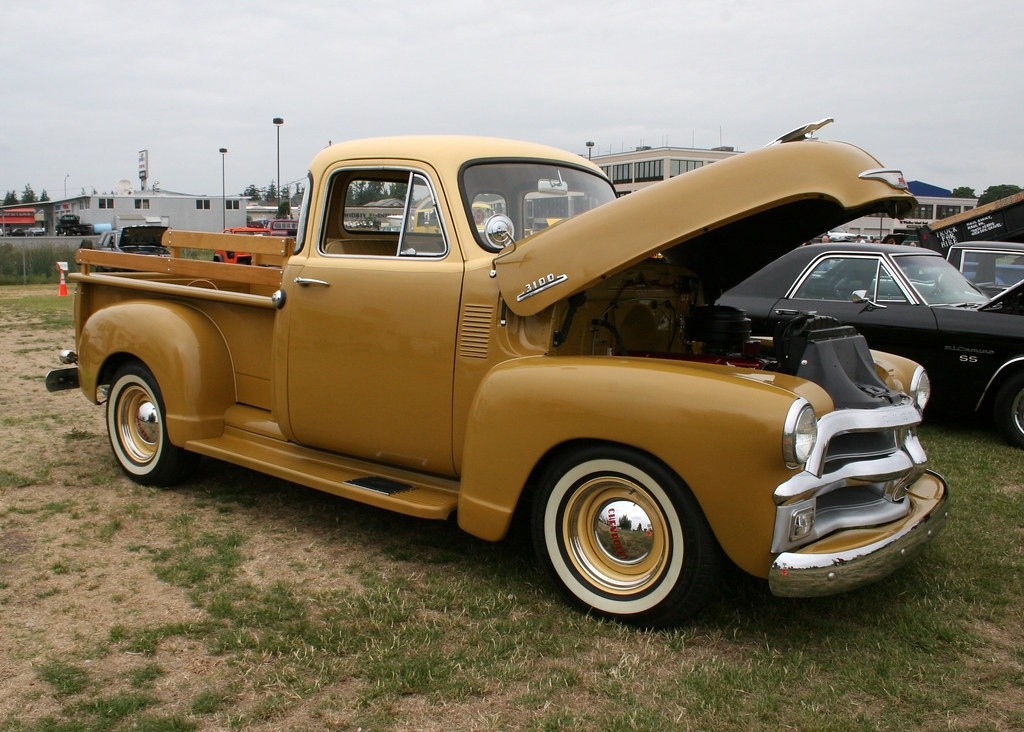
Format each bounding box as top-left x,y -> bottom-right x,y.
414,202 -> 496,235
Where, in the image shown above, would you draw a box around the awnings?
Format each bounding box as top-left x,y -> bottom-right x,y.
1,200 -> 54,207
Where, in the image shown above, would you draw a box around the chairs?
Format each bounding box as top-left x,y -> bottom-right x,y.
807,278 -> 842,301
325,239 -> 410,257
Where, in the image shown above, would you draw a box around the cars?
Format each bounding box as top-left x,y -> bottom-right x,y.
0,226 -> 45,236
806,230 -> 1024,296
712,242 -> 1024,449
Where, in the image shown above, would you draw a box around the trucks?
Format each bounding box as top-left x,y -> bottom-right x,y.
915,189 -> 1024,260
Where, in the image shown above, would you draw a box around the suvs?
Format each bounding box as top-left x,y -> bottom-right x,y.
94,224 -> 169,273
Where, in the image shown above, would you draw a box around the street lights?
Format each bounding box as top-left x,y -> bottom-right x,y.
272,118 -> 284,210
586,142 -> 595,161
219,147 -> 228,230
64,174 -> 69,198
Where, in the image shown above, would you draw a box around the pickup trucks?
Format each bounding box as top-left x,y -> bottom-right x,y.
263,219 -> 298,237
55,214 -> 92,236
213,226 -> 273,266
42,117 -> 951,626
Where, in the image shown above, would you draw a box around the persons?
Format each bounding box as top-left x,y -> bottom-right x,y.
882,235 -> 895,245
855,234 -> 865,243
910,242 -> 916,247
872,238 -> 880,243
821,235 -> 829,243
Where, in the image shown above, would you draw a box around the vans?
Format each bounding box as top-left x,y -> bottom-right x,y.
247,218 -> 268,228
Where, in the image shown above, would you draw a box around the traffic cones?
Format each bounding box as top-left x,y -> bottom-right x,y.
58,270 -> 68,297
